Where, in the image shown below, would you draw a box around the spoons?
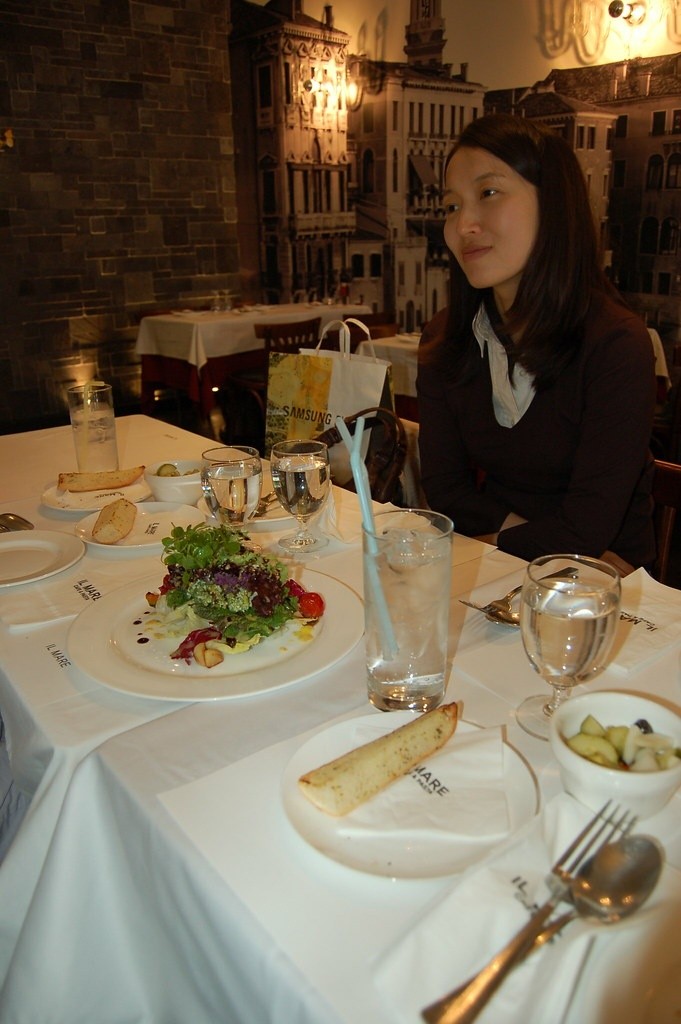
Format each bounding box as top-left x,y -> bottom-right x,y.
421,836 -> 660,1024
485,574 -> 578,626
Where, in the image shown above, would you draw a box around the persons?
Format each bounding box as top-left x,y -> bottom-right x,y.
414,113 -> 657,578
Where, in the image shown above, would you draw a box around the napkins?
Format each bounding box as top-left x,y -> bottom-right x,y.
335,726 -> 511,844
316,480 -> 402,547
451,532 -> 499,569
0,571 -> 108,632
602,567 -> 681,672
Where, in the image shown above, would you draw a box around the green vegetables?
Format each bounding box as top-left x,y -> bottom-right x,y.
159,522 -> 301,644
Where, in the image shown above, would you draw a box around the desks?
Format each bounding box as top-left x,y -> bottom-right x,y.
135,304 -> 373,431
0,412 -> 681,1024
353,327 -> 673,425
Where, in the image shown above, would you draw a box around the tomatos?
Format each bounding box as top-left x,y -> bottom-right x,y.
300,593 -> 324,620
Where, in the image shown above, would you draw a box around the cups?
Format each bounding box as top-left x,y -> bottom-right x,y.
362,510 -> 452,713
67,384 -> 118,474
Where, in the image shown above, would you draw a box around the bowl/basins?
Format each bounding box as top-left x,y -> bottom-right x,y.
142,459 -> 207,505
547,691 -> 680,821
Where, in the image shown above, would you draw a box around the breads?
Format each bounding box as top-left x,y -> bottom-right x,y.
57,465 -> 146,491
91,498 -> 137,544
297,701 -> 459,816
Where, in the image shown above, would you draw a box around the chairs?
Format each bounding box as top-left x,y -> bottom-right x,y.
226,316 -> 321,441
343,311 -> 396,327
327,323 -> 400,354
285,407 -> 408,502
648,459 -> 681,583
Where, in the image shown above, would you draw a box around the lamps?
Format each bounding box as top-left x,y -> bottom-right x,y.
303,77 -> 323,94
607,0 -> 648,26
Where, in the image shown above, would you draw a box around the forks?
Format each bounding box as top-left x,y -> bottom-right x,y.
458,566 -> 579,613
426,800 -> 640,1024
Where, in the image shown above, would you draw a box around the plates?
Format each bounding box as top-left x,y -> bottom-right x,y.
0,530 -> 85,588
74,501 -> 206,550
281,711 -> 539,880
66,567 -> 364,702
40,477 -> 150,513
197,479 -> 293,522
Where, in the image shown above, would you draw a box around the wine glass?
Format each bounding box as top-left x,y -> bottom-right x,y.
516,551 -> 622,739
211,289 -> 233,313
271,439 -> 330,552
201,445 -> 263,555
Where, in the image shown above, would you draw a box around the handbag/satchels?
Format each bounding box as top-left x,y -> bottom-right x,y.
289,407 -> 416,508
266,318 -> 419,508
